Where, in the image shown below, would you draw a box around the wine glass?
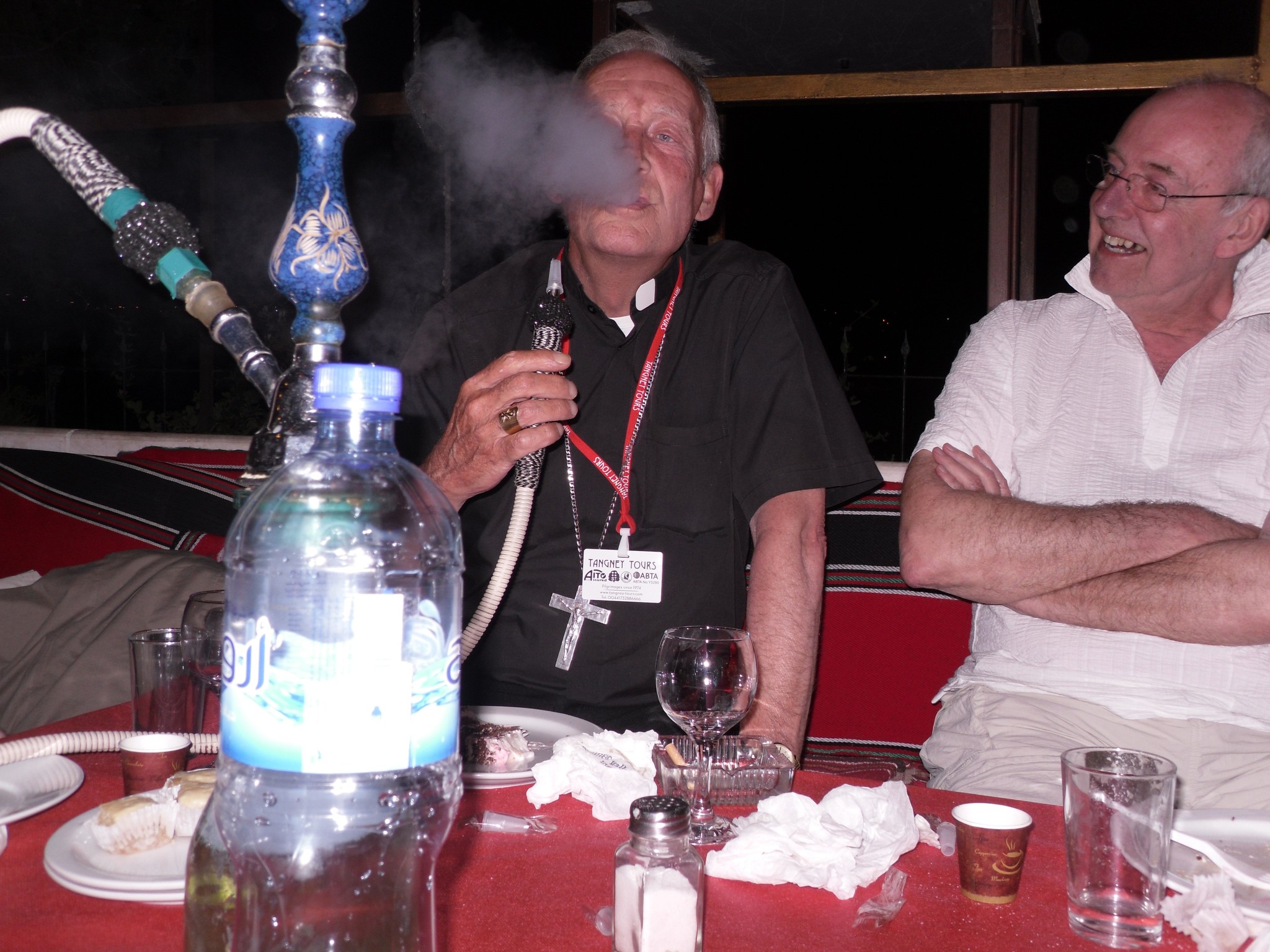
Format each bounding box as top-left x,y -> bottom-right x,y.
654,625 -> 760,845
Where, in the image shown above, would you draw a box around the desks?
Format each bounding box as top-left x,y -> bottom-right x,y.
0,668 -> 1250,952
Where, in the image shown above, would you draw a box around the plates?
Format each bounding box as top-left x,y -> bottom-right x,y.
1117,808 -> 1270,921
43,786 -> 190,905
1,755 -> 84,824
458,706 -> 603,789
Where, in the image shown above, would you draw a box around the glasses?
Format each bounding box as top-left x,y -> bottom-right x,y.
1081,155 -> 1242,213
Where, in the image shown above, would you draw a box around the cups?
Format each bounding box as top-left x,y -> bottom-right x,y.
1060,747 -> 1178,949
131,628 -> 207,755
120,735 -> 192,795
951,802 -> 1033,905
179,589 -> 224,697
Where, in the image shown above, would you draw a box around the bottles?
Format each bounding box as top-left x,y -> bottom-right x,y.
611,795 -> 706,952
185,365 -> 466,950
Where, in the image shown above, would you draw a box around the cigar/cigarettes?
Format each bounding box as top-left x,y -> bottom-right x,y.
665,743 -> 695,790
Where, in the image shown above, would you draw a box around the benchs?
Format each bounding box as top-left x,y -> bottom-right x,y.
0,422 -> 974,792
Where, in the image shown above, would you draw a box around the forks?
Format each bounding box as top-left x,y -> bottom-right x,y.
1093,792 -> 1270,891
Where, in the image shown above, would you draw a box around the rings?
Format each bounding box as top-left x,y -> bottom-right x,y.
498,406 -> 524,435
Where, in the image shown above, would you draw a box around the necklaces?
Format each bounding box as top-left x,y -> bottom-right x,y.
550,330 -> 665,671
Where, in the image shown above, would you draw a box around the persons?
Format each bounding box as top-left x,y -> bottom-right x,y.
340,28 -> 886,770
559,597 -> 603,661
899,70 -> 1270,813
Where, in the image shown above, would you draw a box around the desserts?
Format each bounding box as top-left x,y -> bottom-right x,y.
460,711 -> 536,767
159,768 -> 216,837
91,792 -> 175,854
